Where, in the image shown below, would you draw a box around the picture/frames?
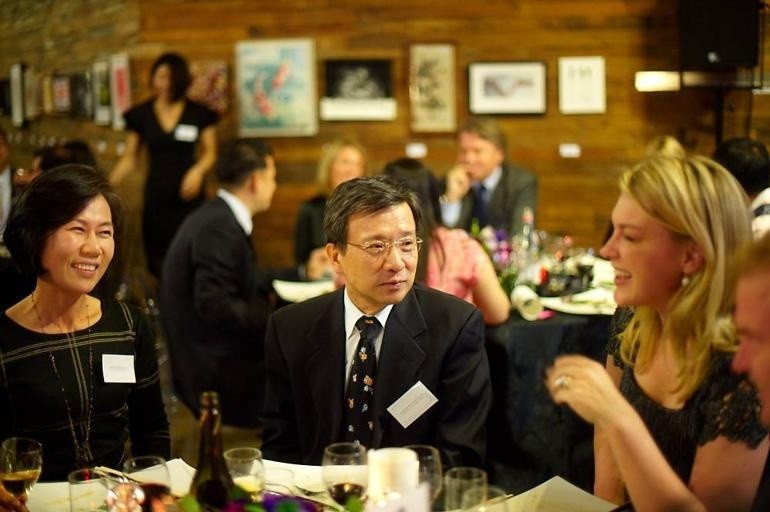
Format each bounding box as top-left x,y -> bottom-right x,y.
9,34 -> 609,142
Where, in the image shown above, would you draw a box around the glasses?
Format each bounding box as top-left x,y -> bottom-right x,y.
344,234 -> 423,256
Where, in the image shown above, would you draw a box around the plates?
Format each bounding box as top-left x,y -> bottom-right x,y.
269,277 -> 337,302
249,458 -> 370,512
27,458 -> 197,512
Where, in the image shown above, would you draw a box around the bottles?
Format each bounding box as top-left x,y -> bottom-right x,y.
187,390 -> 236,512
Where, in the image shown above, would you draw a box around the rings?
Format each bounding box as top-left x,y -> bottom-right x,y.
557,376 -> 570,387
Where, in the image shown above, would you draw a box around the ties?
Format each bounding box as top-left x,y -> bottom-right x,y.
474,185 -> 487,226
344,314 -> 383,451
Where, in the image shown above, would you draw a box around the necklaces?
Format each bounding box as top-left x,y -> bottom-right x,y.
31,291 -> 94,468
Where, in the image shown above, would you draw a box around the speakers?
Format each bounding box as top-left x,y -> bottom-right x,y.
678,0 -> 761,69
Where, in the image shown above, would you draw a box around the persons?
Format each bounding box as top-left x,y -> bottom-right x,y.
729,230 -> 770,428
545,155 -> 770,512
259,177 -> 494,479
159,140 -> 270,428
0,164 -> 171,510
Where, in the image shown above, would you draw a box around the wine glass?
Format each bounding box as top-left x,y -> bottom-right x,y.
472,218 -> 620,320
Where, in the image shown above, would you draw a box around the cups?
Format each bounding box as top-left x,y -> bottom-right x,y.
124,456 -> 172,512
460,487 -> 510,512
443,468 -> 489,511
67,469 -> 110,512
405,444 -> 445,508
224,446 -> 264,501
323,443 -> 370,506
0,437 -> 44,506
